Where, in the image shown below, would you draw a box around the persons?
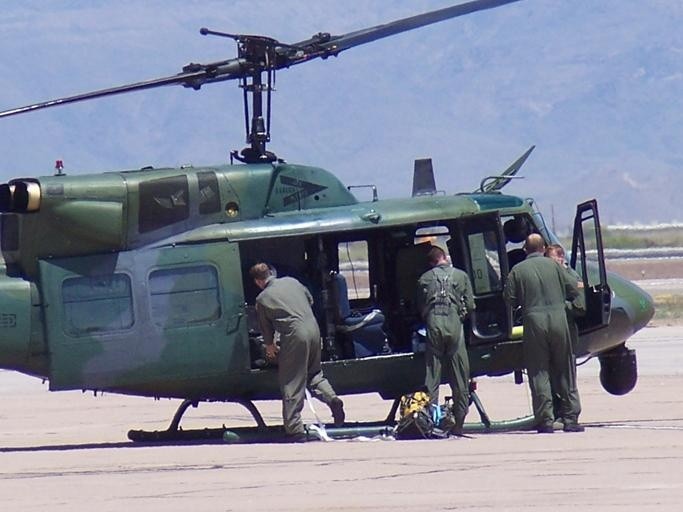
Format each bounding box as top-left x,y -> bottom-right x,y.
501,232 -> 589,434
412,244 -> 478,438
248,261 -> 345,443
546,241 -> 588,421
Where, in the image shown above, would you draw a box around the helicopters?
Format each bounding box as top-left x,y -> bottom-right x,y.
0,0 -> 662,443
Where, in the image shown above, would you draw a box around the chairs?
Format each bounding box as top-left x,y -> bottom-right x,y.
389,240 -> 433,344
447,237 -> 505,290
328,273 -> 388,358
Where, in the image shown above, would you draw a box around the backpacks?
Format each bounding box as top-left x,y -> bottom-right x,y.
425,269 -> 464,347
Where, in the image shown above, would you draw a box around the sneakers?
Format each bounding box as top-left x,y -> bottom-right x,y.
564,423 -> 584,432
287,432 -> 307,441
538,420 -> 554,432
331,398 -> 345,427
453,426 -> 463,435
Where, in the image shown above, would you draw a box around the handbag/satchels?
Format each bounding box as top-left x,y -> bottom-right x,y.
391,390 -> 449,438
337,309 -> 392,357
